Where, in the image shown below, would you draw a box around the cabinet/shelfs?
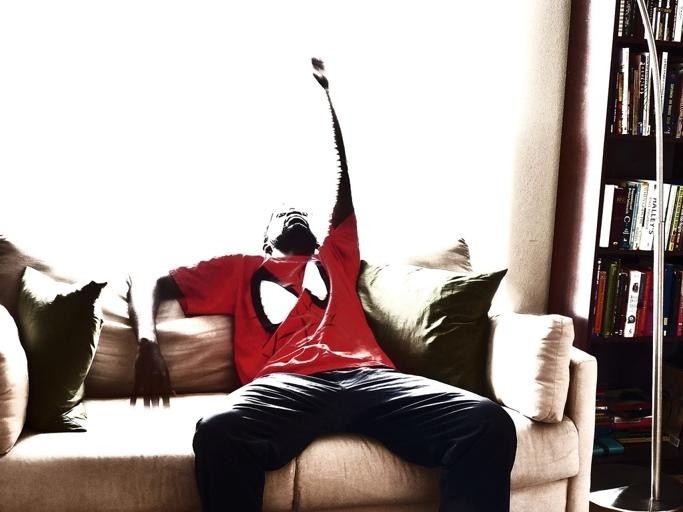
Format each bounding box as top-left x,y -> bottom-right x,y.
549,0 -> 683,465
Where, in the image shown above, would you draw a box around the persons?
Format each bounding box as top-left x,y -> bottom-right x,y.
127,56 -> 518,512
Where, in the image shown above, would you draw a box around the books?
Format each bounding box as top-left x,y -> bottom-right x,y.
593,1 -> 682,457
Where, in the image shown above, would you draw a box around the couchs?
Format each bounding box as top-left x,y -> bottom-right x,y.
0,300 -> 602,512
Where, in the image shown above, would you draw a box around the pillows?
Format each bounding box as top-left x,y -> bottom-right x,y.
10,264 -> 106,433
355,259 -> 508,399
